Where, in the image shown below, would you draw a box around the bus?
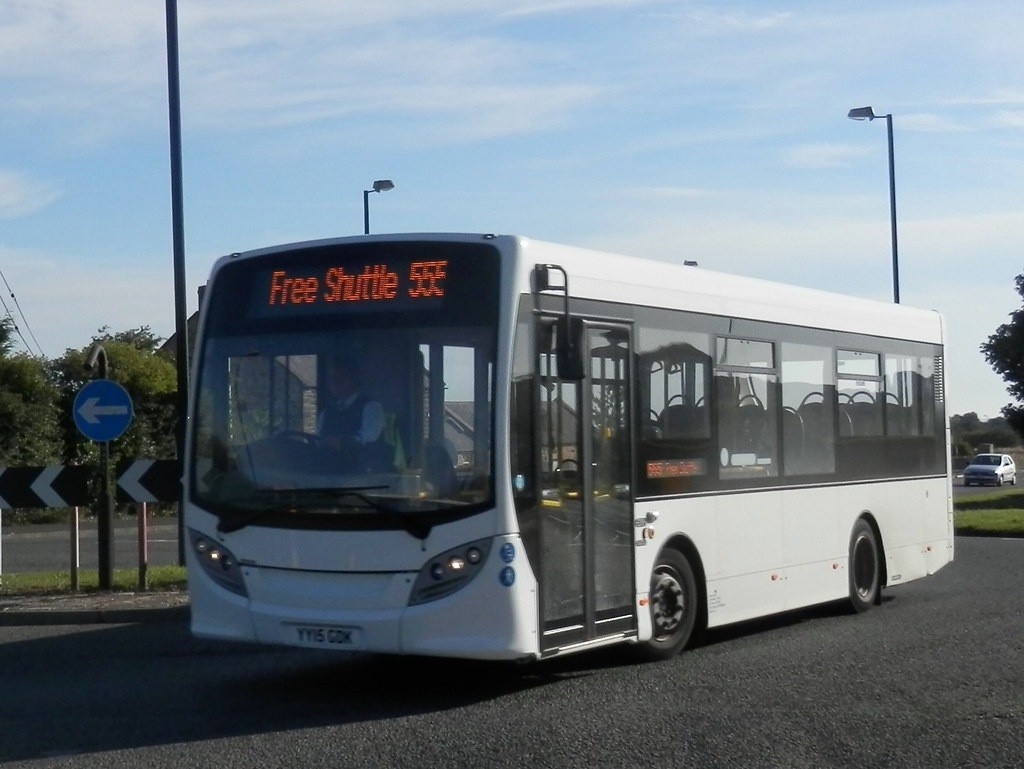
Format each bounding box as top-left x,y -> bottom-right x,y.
177,229 -> 958,665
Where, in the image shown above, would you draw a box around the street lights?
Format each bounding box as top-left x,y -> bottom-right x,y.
363,178 -> 394,233
847,105 -> 905,410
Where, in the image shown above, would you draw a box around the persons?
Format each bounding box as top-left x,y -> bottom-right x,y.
309,360 -> 396,465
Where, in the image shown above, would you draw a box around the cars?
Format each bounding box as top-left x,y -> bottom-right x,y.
962,453 -> 1017,487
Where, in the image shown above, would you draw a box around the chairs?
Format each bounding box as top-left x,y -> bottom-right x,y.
645,391 -> 908,450
554,458 -> 580,494
